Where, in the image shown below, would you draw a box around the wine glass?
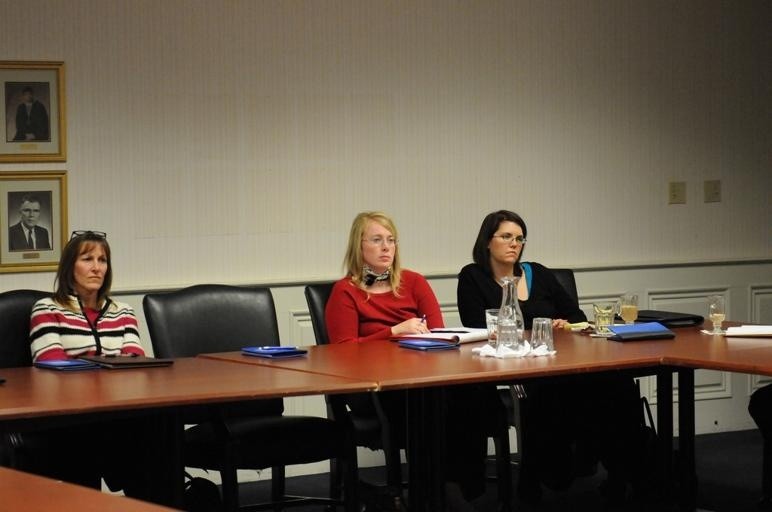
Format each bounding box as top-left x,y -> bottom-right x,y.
707,294 -> 725,335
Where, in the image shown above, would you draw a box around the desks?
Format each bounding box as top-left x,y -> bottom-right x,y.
0,319 -> 378,512
199,319 -> 770,510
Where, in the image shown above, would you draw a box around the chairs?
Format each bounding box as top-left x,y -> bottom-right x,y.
1,290 -> 56,368
496,270 -> 657,472
143,284 -> 358,512
304,281 -> 511,512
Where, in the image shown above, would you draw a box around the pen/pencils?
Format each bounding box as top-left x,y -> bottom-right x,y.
262,346 -> 297,350
105,353 -> 140,357
431,331 -> 471,333
589,334 -> 613,338
421,314 -> 426,323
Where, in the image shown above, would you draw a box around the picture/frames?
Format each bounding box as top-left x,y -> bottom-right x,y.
0,59 -> 67,163
0,169 -> 68,274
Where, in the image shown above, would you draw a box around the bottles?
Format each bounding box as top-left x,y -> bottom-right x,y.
497,275 -> 525,346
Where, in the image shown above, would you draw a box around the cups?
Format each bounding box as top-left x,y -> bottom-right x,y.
617,295 -> 639,324
531,318 -> 554,355
495,320 -> 519,352
592,301 -> 617,335
485,309 -> 504,347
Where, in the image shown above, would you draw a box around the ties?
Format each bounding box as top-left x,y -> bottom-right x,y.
28,229 -> 34,249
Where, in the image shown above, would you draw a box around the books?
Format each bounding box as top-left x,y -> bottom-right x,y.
724,322 -> 772,338
396,337 -> 461,352
606,322 -> 679,342
386,325 -> 495,346
239,345 -> 308,359
33,358 -> 102,372
79,353 -> 175,370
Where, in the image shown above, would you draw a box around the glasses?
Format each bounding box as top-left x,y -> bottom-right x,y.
361,237 -> 397,247
493,234 -> 527,244
71,230 -> 106,238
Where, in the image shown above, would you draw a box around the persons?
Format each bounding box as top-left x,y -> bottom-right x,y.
12,86 -> 51,141
29,233 -> 189,509
9,194 -> 51,252
324,213 -> 498,512
456,211 -> 657,510
